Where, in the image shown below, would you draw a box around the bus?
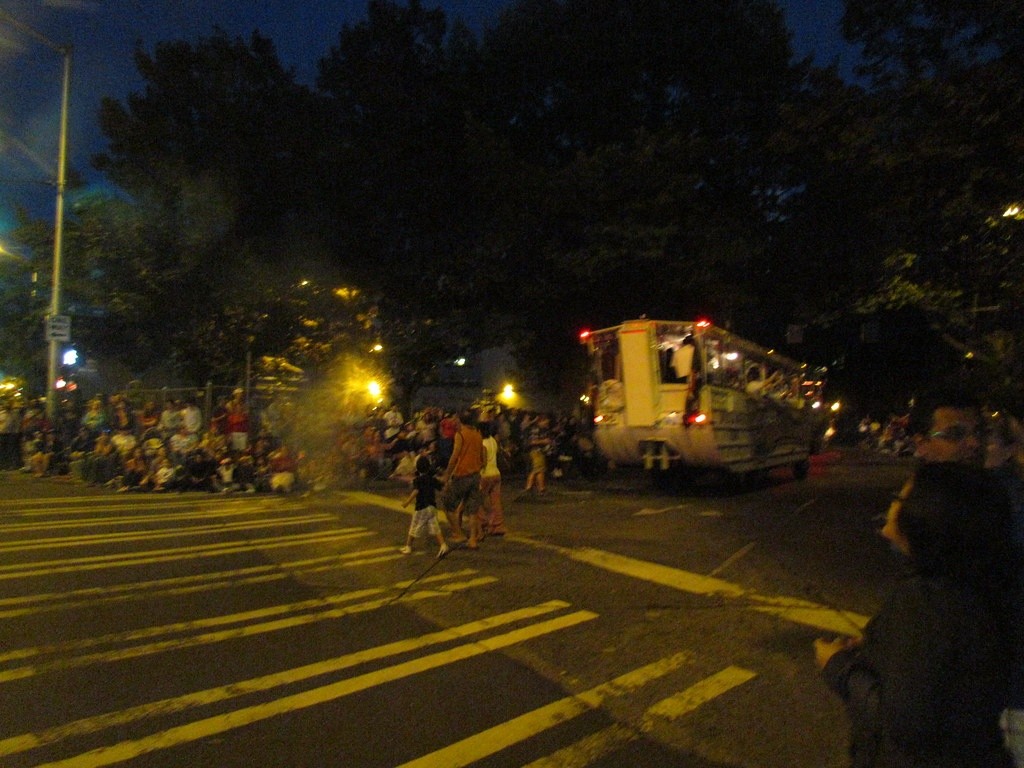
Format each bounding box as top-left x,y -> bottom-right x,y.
580,314 -> 820,487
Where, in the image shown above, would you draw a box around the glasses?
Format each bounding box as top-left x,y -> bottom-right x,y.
887,486 -> 905,503
921,424 -> 988,445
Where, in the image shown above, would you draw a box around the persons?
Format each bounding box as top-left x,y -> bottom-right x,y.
816,394 -> 1024,768
595,337 -> 823,410
0,385 -> 611,556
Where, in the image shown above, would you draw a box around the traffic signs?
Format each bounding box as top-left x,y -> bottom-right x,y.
46,315 -> 72,341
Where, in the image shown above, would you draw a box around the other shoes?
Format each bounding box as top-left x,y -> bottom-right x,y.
435,534 -> 466,548
434,543 -> 450,560
488,531 -> 505,537
475,532 -> 486,542
399,544 -> 412,554
450,539 -> 478,551
